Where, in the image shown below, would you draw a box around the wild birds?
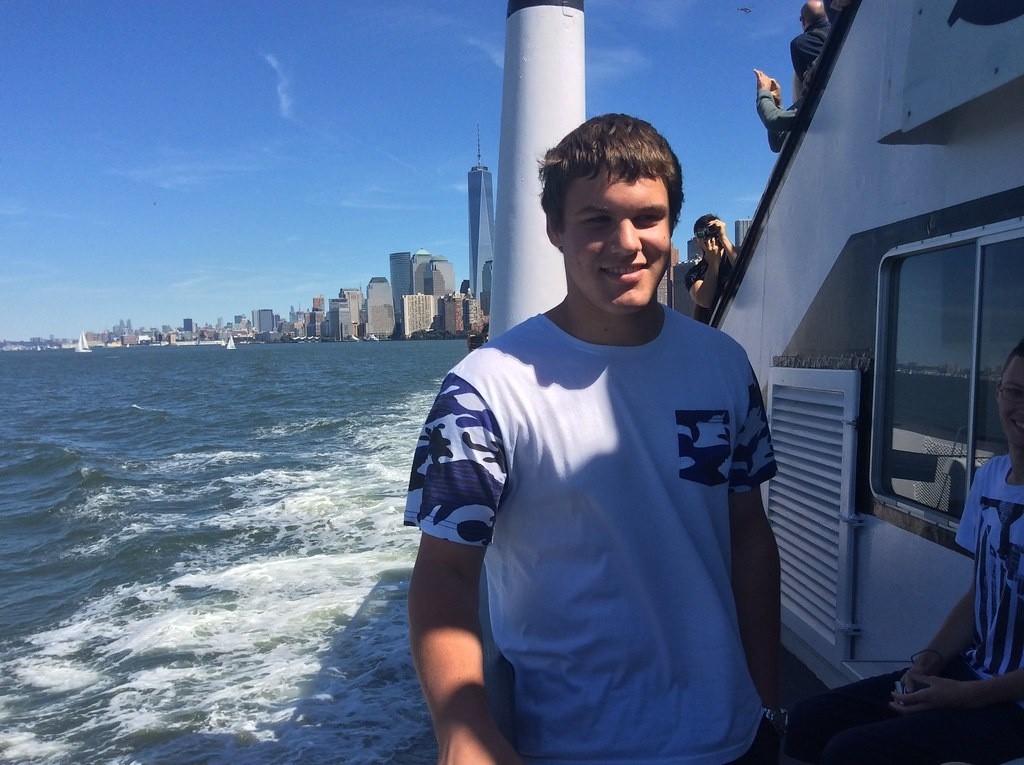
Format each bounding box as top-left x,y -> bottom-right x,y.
737,6 -> 754,15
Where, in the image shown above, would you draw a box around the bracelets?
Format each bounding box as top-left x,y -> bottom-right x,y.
911,649 -> 944,667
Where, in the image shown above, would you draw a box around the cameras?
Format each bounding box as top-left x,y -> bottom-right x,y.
697,225 -> 719,239
896,681 -> 929,705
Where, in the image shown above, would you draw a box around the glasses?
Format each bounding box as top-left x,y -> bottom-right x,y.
997,381 -> 1024,404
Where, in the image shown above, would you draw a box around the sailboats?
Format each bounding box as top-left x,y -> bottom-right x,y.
226,334 -> 237,350
75,330 -> 93,353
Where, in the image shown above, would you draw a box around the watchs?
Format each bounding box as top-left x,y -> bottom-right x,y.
759,705 -> 790,738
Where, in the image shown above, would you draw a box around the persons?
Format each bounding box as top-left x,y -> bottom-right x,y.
783,338 -> 1024,764
400,111 -> 783,765
790,0 -> 832,84
753,68 -> 804,154
467,316 -> 489,352
822,0 -> 852,24
683,214 -> 743,326
